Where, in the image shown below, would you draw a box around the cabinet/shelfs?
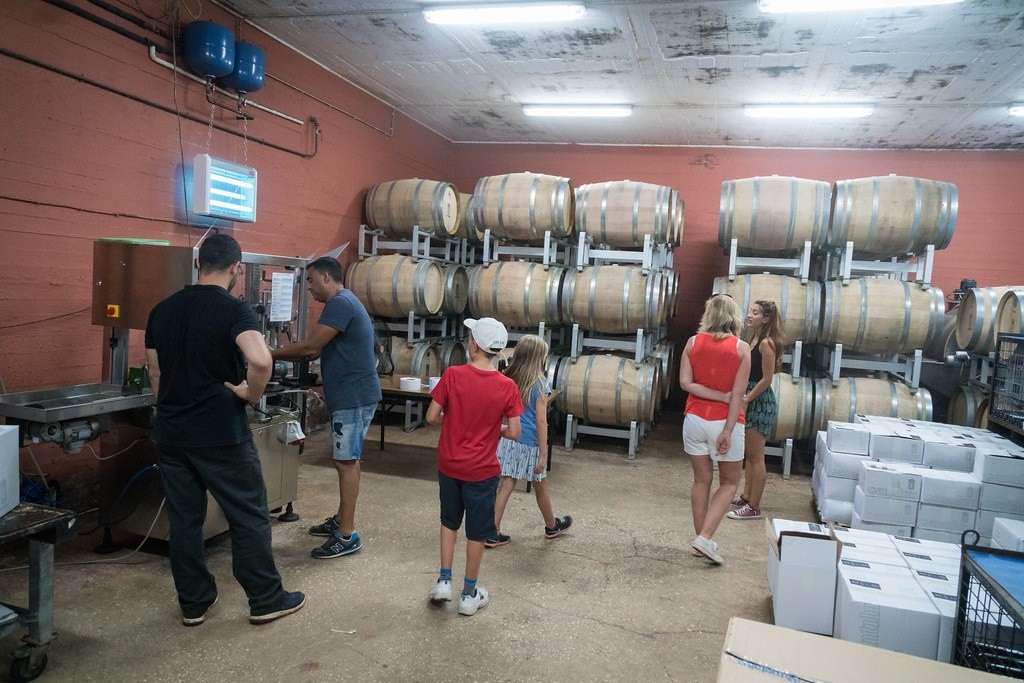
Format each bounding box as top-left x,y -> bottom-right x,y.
357,225 -> 1024,479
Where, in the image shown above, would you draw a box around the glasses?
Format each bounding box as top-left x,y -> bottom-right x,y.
711,294 -> 732,299
237,263 -> 245,275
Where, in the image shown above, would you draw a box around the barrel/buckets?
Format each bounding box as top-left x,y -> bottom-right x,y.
947,384 -> 986,428
827,176 -> 958,253
345,255 -> 444,317
652,341 -> 678,399
467,261 -> 567,327
716,176 -> 830,250
808,376 -> 932,440
436,341 -> 466,377
770,372 -> 813,442
473,173 -> 575,240
575,180 -> 684,248
817,278 -> 945,353
437,262 -> 469,315
974,397 -> 1024,447
928,308 -> 959,362
955,286 -> 1023,356
498,348 -> 562,390
454,192 -> 475,239
562,266 -> 680,334
366,179 -> 461,238
554,355 -> 656,426
711,273 -> 821,345
993,290 -> 1024,362
376,336 -> 441,385
582,351 -> 663,415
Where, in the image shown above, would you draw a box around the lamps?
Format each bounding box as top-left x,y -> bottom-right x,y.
194,103 -> 257,221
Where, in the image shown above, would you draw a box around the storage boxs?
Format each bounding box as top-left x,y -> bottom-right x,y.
717,414 -> 1024,683
0,424 -> 20,518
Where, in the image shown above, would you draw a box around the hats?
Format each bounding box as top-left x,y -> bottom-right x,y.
463,317 -> 508,367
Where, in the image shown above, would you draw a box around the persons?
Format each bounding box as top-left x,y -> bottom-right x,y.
726,298 -> 783,519
424,316 -> 526,617
265,256 -> 384,560
144,234 -> 306,626
679,293 -> 751,564
483,333 -> 573,547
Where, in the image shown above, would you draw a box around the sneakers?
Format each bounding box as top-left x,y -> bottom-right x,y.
727,503 -> 761,519
693,541 -> 718,557
311,530 -> 362,558
428,578 -> 452,602
182,591 -> 219,624
545,515 -> 572,538
691,536 -> 725,564
457,585 -> 488,615
484,533 -> 511,548
730,494 -> 749,507
309,515 -> 339,536
248,591 -> 306,620
